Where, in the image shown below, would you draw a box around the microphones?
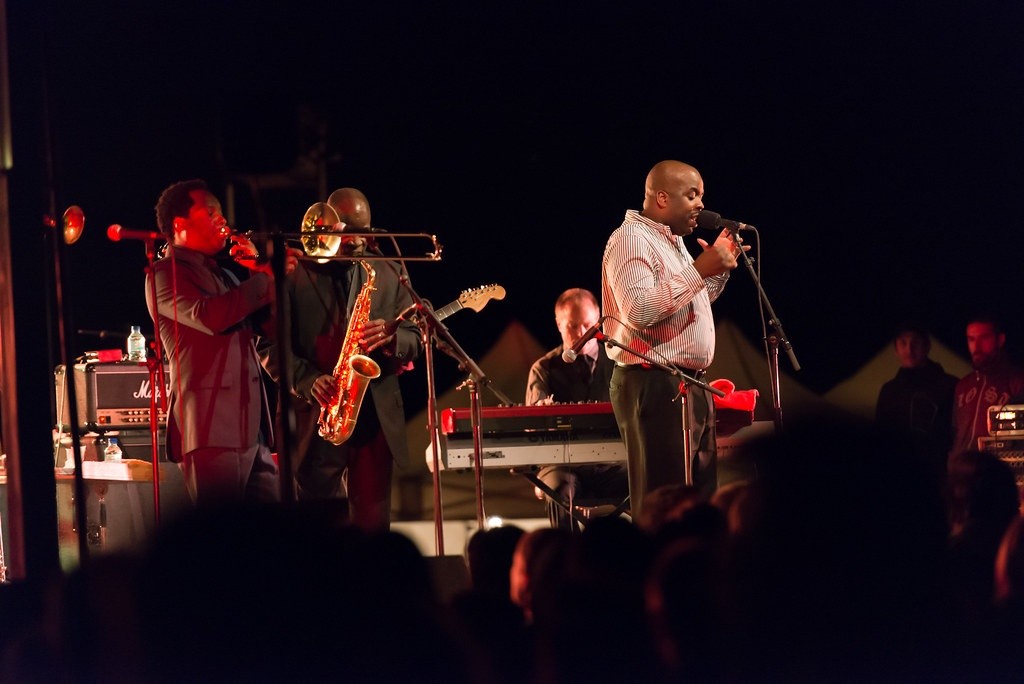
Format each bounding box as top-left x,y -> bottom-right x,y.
107,224 -> 167,242
563,316 -> 608,363
332,222 -> 388,234
696,210 -> 755,231
382,304 -> 417,336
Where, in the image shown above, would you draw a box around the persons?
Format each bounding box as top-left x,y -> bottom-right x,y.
526,288 -> 627,528
144,181 -> 273,504
878,318 -> 1024,512
601,159 -> 751,527
251,189 -> 423,532
0,394 -> 1024,684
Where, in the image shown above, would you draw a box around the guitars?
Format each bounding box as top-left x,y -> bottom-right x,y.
315,282 -> 506,351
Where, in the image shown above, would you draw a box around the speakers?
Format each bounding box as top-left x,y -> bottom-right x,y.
51,432 -> 168,463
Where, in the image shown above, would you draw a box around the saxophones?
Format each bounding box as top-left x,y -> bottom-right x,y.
315,254 -> 383,448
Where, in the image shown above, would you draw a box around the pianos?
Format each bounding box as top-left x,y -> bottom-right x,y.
439,400 -> 756,440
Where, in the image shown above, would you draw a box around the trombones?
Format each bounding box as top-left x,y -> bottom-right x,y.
231,224 -> 442,262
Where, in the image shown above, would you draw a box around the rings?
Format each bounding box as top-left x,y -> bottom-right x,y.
378,332 -> 384,340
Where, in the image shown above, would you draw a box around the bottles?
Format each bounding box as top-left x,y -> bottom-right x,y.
104,438 -> 123,463
127,326 -> 147,362
